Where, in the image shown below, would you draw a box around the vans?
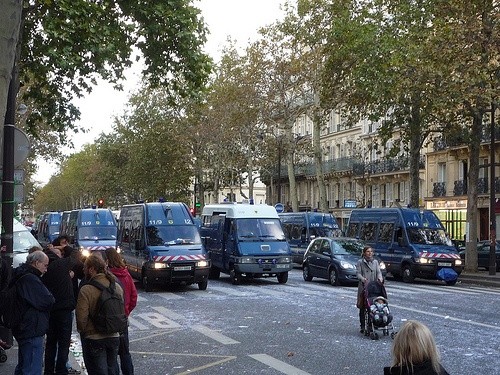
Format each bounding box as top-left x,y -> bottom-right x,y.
60,210 -> 71,239
203,204 -> 293,283
117,199 -> 209,289
346,207 -> 462,285
40,211 -> 60,244
14,216 -> 44,270
69,205 -> 117,251
278,212 -> 345,264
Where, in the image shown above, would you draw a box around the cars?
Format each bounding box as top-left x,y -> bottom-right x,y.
459,239 -> 500,269
303,237 -> 387,284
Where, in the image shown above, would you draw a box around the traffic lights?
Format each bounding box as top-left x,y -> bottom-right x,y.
97,196 -> 105,209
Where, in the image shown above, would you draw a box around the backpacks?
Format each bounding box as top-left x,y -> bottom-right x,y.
0,269 -> 31,329
80,275 -> 127,335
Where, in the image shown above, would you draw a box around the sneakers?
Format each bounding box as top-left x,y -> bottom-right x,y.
360,326 -> 365,333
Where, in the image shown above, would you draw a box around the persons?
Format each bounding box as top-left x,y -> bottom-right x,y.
371,296 -> 390,322
2,251 -> 55,375
384,319 -> 451,375
76,255 -> 126,375
39,248 -> 82,375
356,245 -> 383,333
1,234 -> 86,349
101,247 -> 137,375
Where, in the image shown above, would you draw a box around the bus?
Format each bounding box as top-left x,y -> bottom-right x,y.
34,214 -> 39,244
36,213 -> 43,243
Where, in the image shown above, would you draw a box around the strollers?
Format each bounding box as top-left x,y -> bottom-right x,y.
362,281 -> 399,340
